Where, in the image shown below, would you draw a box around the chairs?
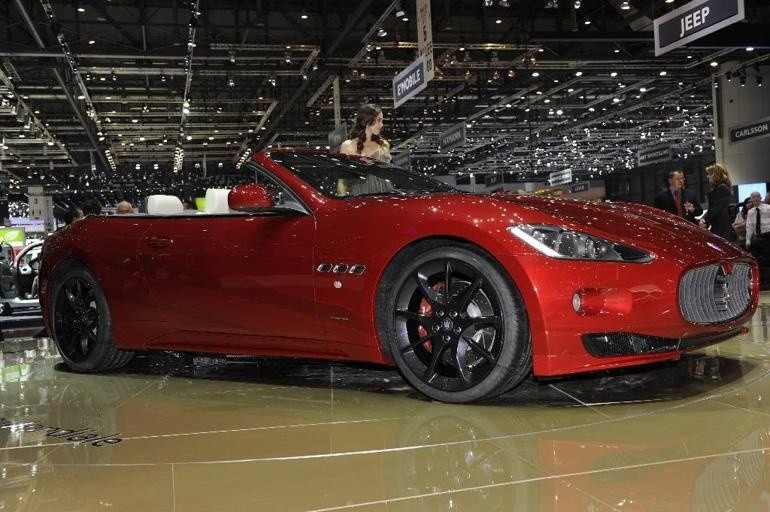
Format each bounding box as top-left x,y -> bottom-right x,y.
138,194 -> 185,215
203,187 -> 247,213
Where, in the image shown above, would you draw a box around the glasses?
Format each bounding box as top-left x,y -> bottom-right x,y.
672,177 -> 686,181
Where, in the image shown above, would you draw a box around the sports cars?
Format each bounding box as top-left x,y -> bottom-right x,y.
36,143 -> 761,406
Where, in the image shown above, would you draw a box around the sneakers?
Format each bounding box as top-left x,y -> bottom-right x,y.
759,285 -> 769,291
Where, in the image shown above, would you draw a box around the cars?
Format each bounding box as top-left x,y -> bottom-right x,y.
0,239 -> 45,317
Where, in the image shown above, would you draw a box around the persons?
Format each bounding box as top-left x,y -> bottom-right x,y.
335,103 -> 396,198
652,168 -> 704,227
62,207 -> 85,227
81,198 -> 103,219
731,197 -> 754,241
115,199 -> 135,216
744,191 -> 770,292
183,199 -> 198,211
698,164 -> 737,243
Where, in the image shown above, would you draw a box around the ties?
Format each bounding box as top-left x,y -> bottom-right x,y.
755,207 -> 761,234
673,191 -> 683,217
